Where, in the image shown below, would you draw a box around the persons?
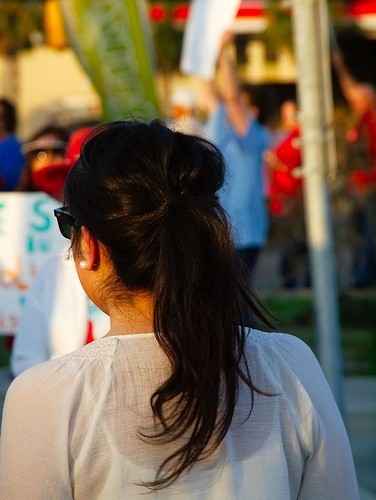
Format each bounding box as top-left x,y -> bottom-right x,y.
0,98 -> 112,378
0,111 -> 359,500
196,30 -> 376,289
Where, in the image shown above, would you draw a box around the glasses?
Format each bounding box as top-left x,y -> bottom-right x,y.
54,206 -> 82,239
